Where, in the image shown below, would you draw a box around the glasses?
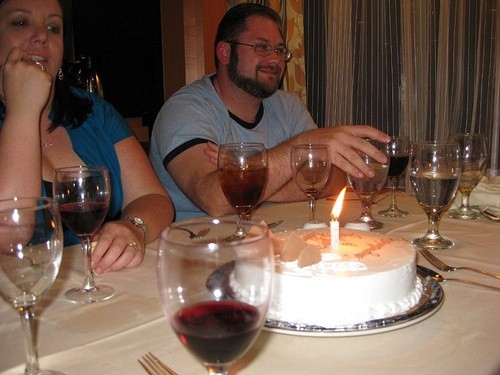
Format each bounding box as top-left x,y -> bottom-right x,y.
227,40 -> 292,62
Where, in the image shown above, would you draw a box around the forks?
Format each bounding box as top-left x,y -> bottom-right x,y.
137,352 -> 180,375
172,227 -> 210,239
419,248 -> 500,279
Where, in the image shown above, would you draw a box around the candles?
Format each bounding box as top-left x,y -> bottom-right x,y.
329,184 -> 348,250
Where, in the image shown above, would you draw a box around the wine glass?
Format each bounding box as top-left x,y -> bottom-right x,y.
157,218 -> 276,375
446,133 -> 488,221
377,136 -> 410,217
217,143 -> 268,243
52,166 -> 116,305
408,141 -> 463,250
0,198 -> 65,374
347,140 -> 390,230
290,144 -> 331,223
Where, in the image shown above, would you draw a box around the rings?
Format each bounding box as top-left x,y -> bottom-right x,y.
35,61 -> 45,68
126,240 -> 140,251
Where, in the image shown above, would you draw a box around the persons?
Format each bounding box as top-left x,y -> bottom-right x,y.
148,2 -> 392,222
0,0 -> 176,275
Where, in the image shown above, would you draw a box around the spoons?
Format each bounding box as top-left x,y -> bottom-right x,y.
416,264 -> 500,292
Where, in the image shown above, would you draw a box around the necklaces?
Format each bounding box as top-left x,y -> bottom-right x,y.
39,136 -> 52,152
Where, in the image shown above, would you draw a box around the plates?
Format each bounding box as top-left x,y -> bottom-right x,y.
160,219 -> 268,245
206,259 -> 446,337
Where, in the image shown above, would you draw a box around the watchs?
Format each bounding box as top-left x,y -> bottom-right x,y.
120,215 -> 148,245
